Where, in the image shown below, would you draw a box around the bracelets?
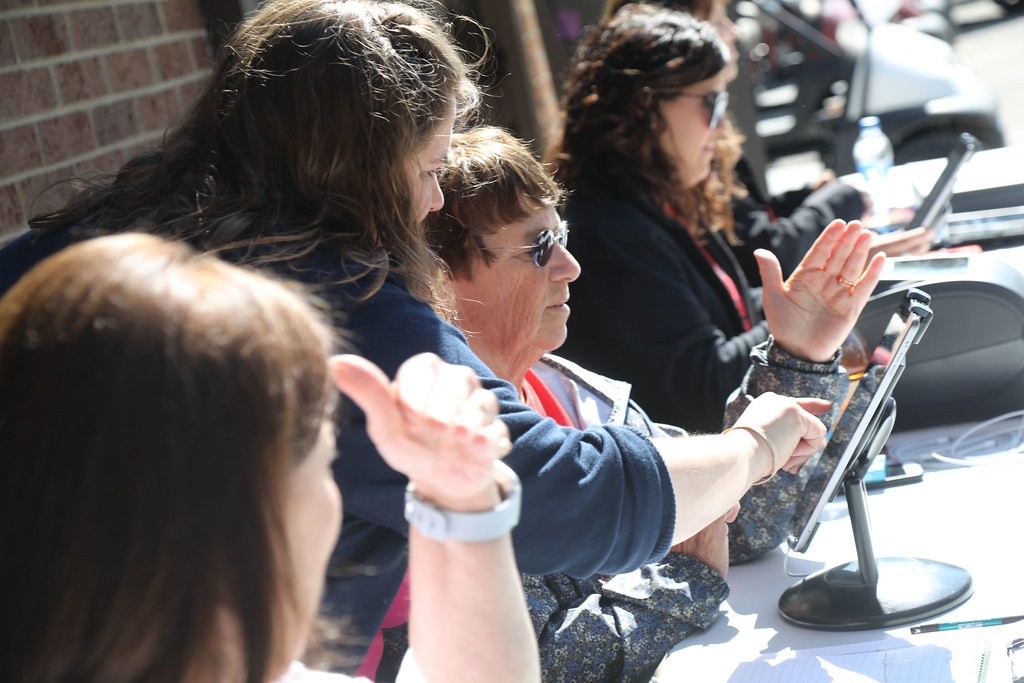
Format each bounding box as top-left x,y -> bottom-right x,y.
722,423 -> 779,486
404,460 -> 522,541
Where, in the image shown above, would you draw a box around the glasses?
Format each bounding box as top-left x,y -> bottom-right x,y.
474,221 -> 571,269
660,88 -> 729,129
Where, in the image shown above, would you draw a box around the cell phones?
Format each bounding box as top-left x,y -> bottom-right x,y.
885,461 -> 922,486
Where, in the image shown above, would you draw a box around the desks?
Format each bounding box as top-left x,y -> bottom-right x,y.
648,415 -> 1024,683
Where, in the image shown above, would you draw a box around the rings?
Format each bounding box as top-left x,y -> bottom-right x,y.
837,275 -> 856,289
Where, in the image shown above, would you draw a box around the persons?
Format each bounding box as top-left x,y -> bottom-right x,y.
0,0 -> 952,683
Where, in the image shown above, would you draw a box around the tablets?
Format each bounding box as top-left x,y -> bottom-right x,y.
907,135 -> 976,233
787,295 -> 923,552
